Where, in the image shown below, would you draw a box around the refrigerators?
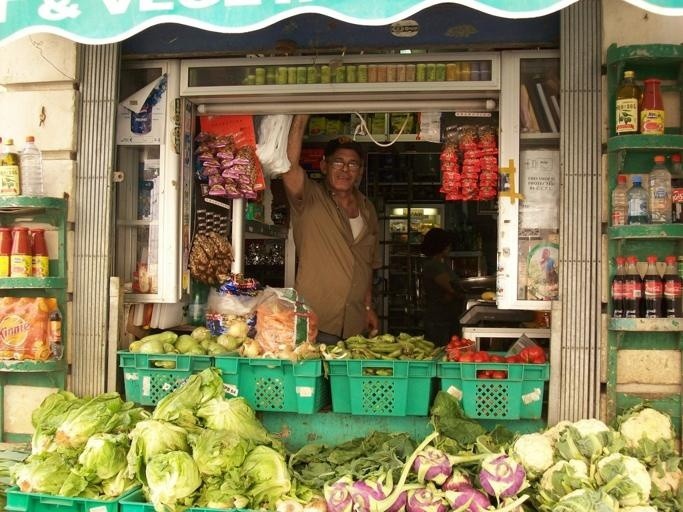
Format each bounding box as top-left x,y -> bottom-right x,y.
383,198 -> 446,331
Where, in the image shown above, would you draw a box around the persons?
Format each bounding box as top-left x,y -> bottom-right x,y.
422,228 -> 465,348
539,248 -> 558,286
281,115 -> 380,348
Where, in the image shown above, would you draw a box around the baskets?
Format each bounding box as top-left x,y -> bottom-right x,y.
322,348 -> 440,417
439,350 -> 552,423
118,350 -> 213,409
119,486 -> 255,511
215,353 -> 328,419
2,487 -> 119,511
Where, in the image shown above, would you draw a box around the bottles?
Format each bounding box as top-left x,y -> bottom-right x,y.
0,226 -> 50,278
615,67 -> 666,137
611,155 -> 682,224
610,253 -> 683,318
20,135 -> 43,195
0,296 -> 64,362
0,138 -> 23,195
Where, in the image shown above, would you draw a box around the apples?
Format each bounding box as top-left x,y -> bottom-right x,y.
130,332 -> 298,369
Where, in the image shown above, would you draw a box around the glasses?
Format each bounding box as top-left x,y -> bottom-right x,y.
328,157 -> 362,172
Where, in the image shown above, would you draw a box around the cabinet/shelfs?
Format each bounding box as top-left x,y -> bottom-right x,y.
606,42 -> 682,428
0,191 -> 70,443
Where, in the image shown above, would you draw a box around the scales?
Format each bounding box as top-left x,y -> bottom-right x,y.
458,299 -> 550,327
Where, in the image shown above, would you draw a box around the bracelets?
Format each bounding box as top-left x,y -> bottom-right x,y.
365,305 -> 374,310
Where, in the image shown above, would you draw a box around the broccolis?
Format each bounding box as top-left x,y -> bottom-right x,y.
513,407 -> 683,512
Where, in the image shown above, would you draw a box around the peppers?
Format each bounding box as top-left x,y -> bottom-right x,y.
336,332 -> 446,376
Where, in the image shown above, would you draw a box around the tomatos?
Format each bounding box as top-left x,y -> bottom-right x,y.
446,335 -> 546,379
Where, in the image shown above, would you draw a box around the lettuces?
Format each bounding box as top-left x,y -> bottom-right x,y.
9,366 -> 292,512
